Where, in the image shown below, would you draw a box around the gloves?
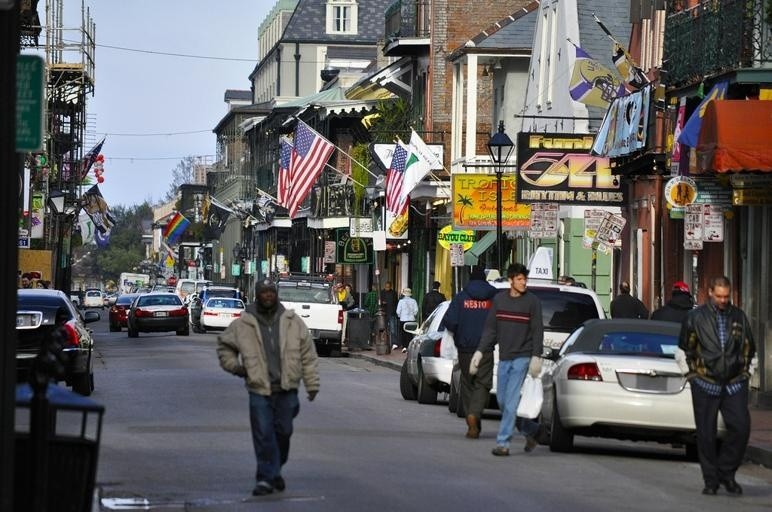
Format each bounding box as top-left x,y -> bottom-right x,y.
529,357 -> 541,378
468,350 -> 483,375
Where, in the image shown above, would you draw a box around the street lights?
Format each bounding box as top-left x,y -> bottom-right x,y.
231,240 -> 242,288
365,182 -> 392,353
46,186 -> 65,289
194,254 -> 200,280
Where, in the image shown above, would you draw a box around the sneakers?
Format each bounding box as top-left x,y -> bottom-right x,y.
526,424 -> 544,452
391,344 -> 399,350
402,347 -> 407,353
493,447 -> 511,456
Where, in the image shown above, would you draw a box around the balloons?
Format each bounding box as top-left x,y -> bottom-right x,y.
94,155 -> 106,183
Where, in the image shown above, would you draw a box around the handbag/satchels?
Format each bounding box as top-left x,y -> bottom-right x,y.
516,374 -> 544,418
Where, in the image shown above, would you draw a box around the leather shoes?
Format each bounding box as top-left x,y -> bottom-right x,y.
274,476 -> 287,492
703,486 -> 719,497
253,482 -> 274,495
722,479 -> 743,498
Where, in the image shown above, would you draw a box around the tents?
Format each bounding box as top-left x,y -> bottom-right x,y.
696,99 -> 772,174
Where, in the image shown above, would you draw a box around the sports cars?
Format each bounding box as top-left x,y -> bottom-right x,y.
536,319 -> 726,462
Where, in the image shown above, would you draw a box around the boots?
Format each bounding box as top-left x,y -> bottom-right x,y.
466,414 -> 480,438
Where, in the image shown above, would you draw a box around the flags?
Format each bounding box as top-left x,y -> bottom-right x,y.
400,129 -> 439,205
594,16 -> 650,90
252,189 -> 279,226
159,251 -> 177,268
77,184 -> 115,233
208,203 -> 231,234
568,41 -> 626,109
677,81 -> 729,148
385,140 -> 410,214
96,226 -> 111,249
277,139 -> 294,208
163,213 -> 192,242
79,220 -> 96,246
83,137 -> 107,180
287,119 -> 335,217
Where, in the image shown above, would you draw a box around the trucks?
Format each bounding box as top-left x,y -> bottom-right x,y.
117,273 -> 150,294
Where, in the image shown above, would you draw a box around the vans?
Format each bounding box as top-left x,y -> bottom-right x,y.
191,286 -> 240,333
176,278 -> 213,326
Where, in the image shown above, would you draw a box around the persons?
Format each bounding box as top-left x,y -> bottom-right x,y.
36,280 -> 51,289
650,282 -> 696,322
47,307 -> 79,348
78,287 -> 86,310
559,275 -> 581,286
240,290 -> 250,306
445,269 -> 497,439
678,276 -> 756,495
396,288 -> 419,352
215,279 -> 322,495
21,273 -> 37,289
422,281 -> 446,322
381,281 -> 398,350
610,282 -> 649,320
336,284 -> 355,346
469,263 -> 543,456
16,274 -> 20,289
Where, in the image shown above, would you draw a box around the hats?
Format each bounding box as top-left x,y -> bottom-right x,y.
401,288 -> 414,296
254,278 -> 278,291
674,281 -> 692,292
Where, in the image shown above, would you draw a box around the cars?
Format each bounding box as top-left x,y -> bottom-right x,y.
448,278 -> 607,434
15,289 -> 94,396
134,287 -> 152,294
86,291 -> 104,312
399,299 -> 459,404
71,291 -> 84,307
200,297 -> 246,334
128,292 -> 189,338
108,293 -> 140,332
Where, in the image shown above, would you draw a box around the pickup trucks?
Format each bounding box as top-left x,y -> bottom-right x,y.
275,271 -> 344,358
